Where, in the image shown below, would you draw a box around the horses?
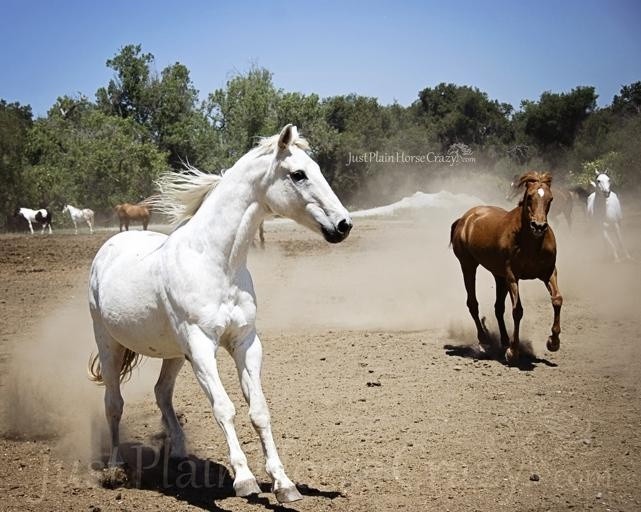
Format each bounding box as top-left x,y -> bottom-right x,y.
13,207 -> 52,235
85,122 -> 354,504
449,170 -> 563,366
572,167 -> 630,263
114,194 -> 156,232
61,204 -> 95,234
505,174 -> 589,232
